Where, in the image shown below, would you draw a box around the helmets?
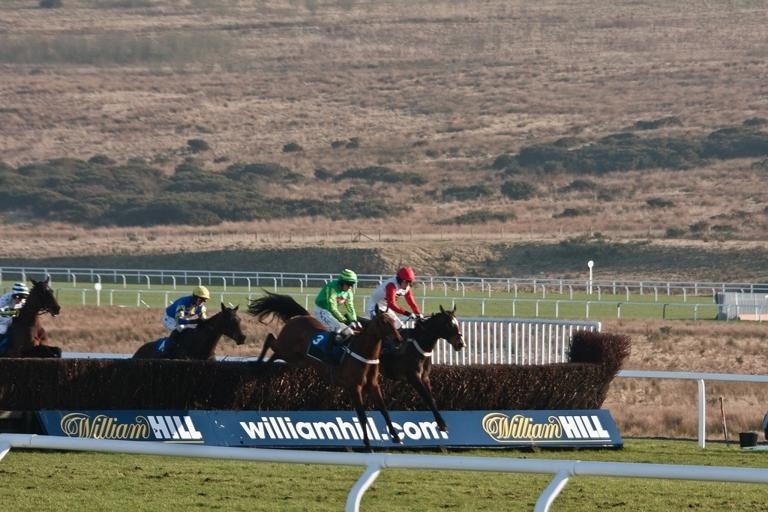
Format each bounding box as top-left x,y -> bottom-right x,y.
338,269 -> 359,283
396,266 -> 416,282
11,282 -> 30,298
192,285 -> 211,299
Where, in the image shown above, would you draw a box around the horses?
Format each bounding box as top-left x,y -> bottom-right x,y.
245,288 -> 404,452
0,275 -> 62,358
132,302 -> 246,360
343,304 -> 463,432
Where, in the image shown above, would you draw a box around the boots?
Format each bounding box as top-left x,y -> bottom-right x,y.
326,332 -> 336,355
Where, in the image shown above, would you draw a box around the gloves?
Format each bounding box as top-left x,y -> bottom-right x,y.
408,313 -> 417,321
356,321 -> 362,329
347,321 -> 357,330
416,313 -> 424,319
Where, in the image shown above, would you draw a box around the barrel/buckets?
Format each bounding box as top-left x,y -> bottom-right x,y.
739,432 -> 757,447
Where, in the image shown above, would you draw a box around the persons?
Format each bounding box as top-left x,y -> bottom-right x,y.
0,282 -> 30,336
311,268 -> 358,337
367,267 -> 424,329
154,285 -> 210,353
